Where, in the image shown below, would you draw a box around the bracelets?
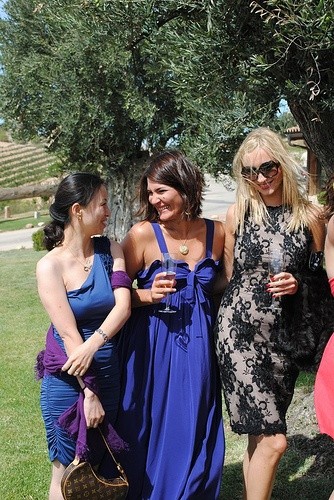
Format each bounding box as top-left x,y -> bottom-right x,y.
94,329 -> 109,347
82,386 -> 87,391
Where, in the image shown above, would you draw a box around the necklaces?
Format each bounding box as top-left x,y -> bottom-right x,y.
62,239 -> 92,271
163,220 -> 194,255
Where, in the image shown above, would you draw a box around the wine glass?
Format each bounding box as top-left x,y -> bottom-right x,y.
263,249 -> 283,310
159,253 -> 178,313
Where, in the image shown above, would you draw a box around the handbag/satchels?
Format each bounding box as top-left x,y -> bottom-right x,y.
60,425 -> 129,500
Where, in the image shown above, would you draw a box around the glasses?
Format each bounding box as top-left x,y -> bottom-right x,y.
241,160 -> 281,181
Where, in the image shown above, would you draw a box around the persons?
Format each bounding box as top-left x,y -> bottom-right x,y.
120,150 -> 226,500
214,128 -> 324,500
36,172 -> 132,500
